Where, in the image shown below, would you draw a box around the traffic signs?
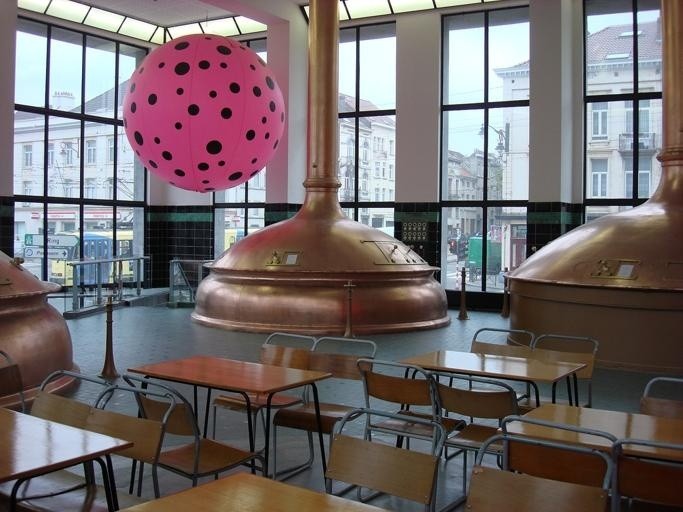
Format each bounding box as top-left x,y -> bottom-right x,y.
24,234 -> 79,248
23,247 -> 67,260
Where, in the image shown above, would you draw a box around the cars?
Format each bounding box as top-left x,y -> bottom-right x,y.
448,233 -> 468,258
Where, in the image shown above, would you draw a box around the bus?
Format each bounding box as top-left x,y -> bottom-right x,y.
51,221 -> 262,289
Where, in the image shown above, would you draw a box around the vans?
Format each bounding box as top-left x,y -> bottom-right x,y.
465,237 -> 501,275
